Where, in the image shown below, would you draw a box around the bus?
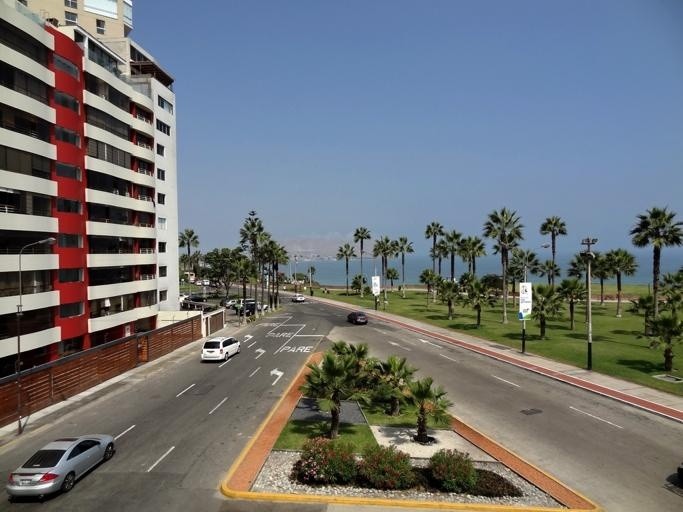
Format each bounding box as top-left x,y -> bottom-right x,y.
184,272 -> 195,283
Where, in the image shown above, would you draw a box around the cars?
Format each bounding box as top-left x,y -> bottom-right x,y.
186,296 -> 207,302
292,294 -> 304,302
196,280 -> 210,286
347,311 -> 368,325
7,433 -> 115,499
220,299 -> 268,316
200,337 -> 240,361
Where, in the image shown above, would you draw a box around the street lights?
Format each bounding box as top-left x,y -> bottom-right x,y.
16,238 -> 57,433
582,237 -> 598,370
522,243 -> 551,351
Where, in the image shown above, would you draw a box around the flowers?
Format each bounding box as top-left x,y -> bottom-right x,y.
296,435 -> 480,494
